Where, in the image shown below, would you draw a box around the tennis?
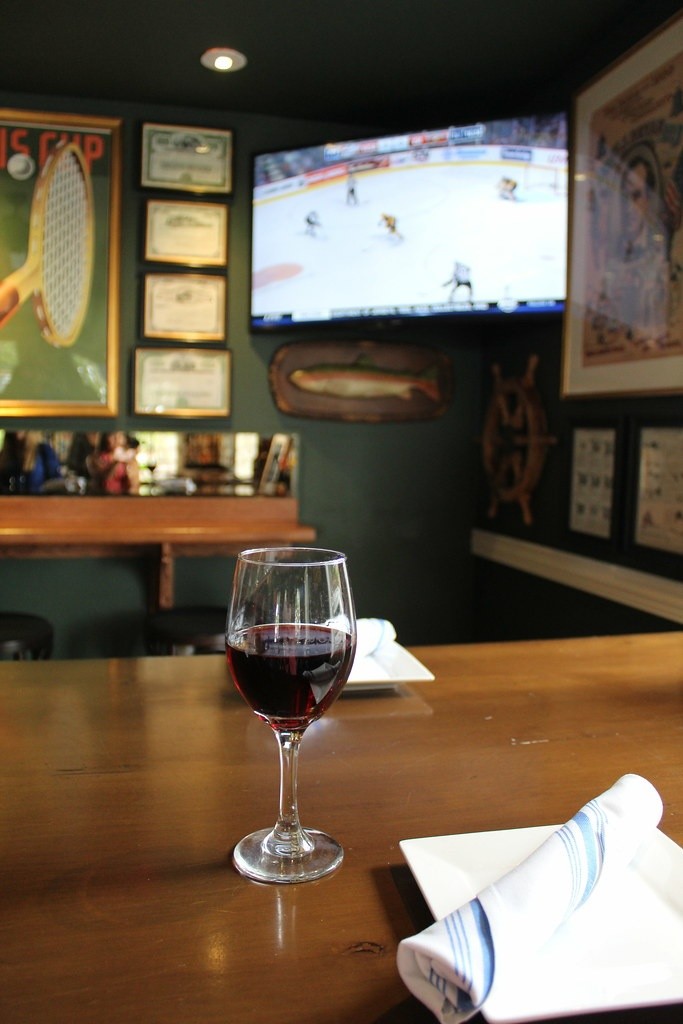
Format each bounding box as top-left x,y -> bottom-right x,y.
7,153 -> 35,181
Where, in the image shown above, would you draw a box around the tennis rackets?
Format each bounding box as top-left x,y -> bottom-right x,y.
0,137 -> 96,350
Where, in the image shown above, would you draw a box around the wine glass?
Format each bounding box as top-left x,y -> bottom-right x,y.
225,548 -> 357,883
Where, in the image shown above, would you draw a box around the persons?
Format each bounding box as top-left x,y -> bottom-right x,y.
346,172 -> 357,205
496,177 -> 516,199
0,430 -> 140,495
305,210 -> 317,228
448,261 -> 472,301
598,158 -> 668,330
380,213 -> 397,233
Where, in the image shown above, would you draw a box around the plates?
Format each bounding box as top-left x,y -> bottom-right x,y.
399,822 -> 683,1024
344,640 -> 437,694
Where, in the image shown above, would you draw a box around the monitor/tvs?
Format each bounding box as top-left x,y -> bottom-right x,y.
248,105 -> 570,334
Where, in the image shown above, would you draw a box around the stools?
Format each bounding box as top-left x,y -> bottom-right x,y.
149,605 -> 226,656
0,611 -> 55,661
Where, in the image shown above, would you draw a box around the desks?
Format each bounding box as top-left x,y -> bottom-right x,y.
0,633 -> 682,1024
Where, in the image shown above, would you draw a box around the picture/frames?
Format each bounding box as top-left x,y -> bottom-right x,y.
137,269 -> 229,345
136,119 -> 236,199
0,108 -> 121,417
139,195 -> 230,269
131,344 -> 232,421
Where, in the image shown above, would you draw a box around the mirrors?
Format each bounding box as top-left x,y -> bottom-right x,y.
558,15 -> 682,397
